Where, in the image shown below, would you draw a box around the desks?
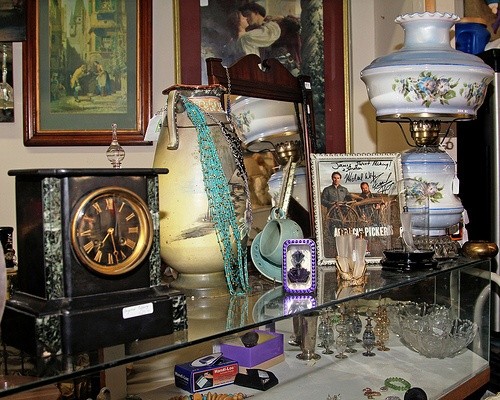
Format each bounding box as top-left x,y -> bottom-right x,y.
0,240 -> 500,400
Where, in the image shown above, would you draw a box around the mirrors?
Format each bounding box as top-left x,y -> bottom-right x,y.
204,51 -> 315,265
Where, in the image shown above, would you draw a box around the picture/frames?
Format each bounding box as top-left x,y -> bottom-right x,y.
173,0 -> 354,155
22,0 -> 155,149
309,149 -> 407,266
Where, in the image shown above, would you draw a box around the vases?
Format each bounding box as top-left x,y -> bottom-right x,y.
358,10 -> 495,122
225,96 -> 301,152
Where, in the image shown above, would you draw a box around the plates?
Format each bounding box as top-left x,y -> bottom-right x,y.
250,231 -> 282,283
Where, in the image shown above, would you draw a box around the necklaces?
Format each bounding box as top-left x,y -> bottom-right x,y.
225,296 -> 248,332
178,95 -> 253,296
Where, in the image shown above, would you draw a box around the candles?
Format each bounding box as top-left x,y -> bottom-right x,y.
336,228 -> 350,273
353,232 -> 367,279
398,204 -> 419,252
347,227 -> 357,269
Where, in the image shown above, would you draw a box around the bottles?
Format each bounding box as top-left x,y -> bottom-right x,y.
288,313 -> 302,346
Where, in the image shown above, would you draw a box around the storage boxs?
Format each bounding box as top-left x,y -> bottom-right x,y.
174,352 -> 240,395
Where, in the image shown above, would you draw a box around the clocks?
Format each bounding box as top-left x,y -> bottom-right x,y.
0,165 -> 188,358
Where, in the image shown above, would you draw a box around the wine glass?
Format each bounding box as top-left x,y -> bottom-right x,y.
295,311 -> 322,361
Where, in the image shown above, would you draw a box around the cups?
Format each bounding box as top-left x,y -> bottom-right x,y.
259,207 -> 304,265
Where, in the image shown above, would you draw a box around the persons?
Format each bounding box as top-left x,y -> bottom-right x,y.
322,172 -> 352,235
356,182 -> 382,226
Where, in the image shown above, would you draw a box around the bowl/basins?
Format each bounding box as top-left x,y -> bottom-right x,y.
399,316 -> 477,358
386,302 -> 447,336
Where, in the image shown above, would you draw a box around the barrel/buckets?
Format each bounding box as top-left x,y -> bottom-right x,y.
455,22 -> 490,55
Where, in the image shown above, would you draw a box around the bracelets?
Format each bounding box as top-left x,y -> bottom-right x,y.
385,377 -> 411,391
167,393 -> 254,400
